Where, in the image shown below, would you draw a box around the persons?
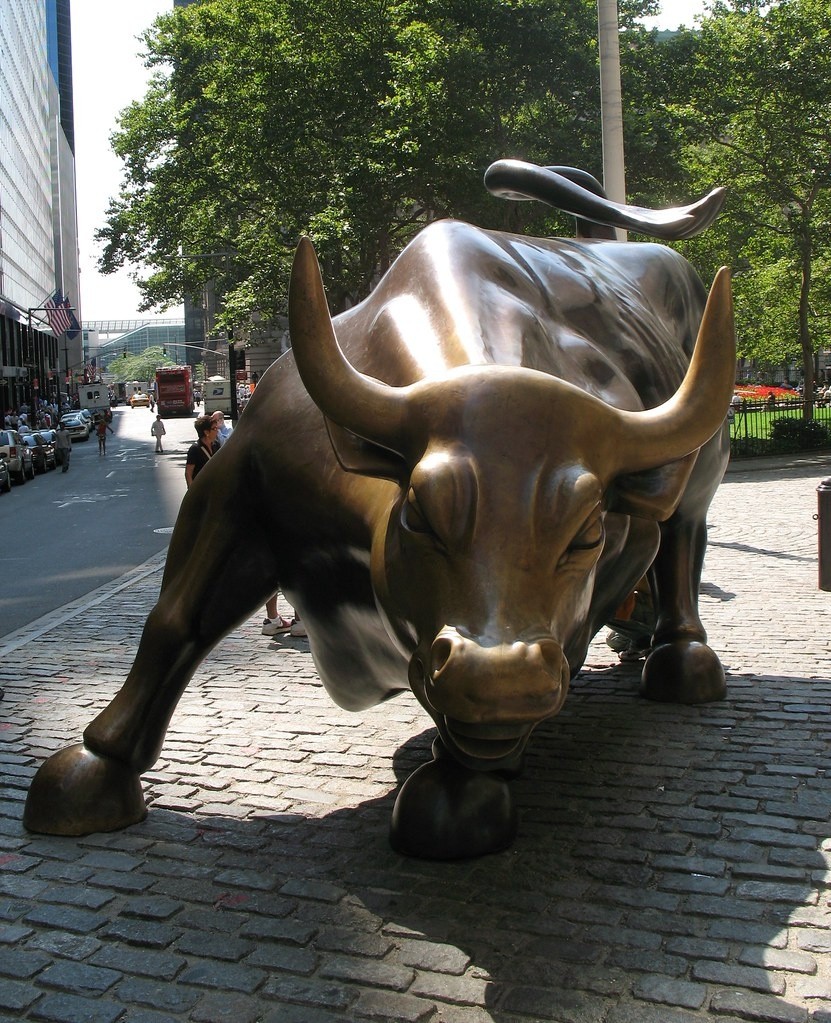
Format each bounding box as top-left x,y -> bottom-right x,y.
262,595 -> 309,635
211,411 -> 232,444
151,415 -> 166,452
745,369 -> 752,379
150,392 -> 155,412
96,419 -> 113,455
731,393 -> 741,409
55,422 -> 72,472
4,394 -> 77,432
185,416 -> 220,485
767,391 -> 775,403
195,391 -> 200,406
799,381 -> 831,407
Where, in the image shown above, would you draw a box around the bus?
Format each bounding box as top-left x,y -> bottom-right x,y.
155,366 -> 195,417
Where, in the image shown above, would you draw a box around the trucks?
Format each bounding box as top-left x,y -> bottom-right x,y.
107,381 -> 147,407
79,384 -> 113,425
202,375 -> 233,419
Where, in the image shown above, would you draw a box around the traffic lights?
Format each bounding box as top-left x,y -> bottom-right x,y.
123,352 -> 126,361
162,349 -> 166,357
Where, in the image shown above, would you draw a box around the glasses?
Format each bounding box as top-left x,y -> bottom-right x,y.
210,426 -> 218,431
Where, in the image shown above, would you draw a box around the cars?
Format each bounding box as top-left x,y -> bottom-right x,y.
56,409 -> 95,443
19,432 -> 56,474
41,432 -> 60,465
125,390 -> 150,409
0,452 -> 10,494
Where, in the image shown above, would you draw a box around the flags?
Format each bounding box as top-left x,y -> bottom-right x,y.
87,358 -> 96,375
65,297 -> 80,339
44,290 -> 71,337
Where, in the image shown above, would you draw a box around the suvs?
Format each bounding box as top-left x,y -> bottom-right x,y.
0,429 -> 35,486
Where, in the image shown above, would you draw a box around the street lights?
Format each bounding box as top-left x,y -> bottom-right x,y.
64,329 -> 95,406
163,252 -> 239,427
28,307 -> 77,425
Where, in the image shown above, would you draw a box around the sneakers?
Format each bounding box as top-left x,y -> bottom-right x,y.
261,614 -> 291,635
290,619 -> 306,635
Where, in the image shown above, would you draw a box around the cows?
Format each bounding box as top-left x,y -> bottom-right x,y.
23,159 -> 737,863
